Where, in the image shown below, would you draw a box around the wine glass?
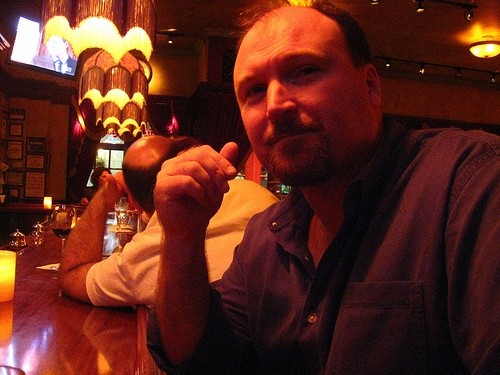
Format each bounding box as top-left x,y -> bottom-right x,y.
52,205 -> 77,270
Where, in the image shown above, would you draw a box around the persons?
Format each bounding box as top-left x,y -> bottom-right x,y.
144,0 -> 500,375
56,131 -> 282,311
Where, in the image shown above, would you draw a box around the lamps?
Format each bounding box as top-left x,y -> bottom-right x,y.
464,7 -> 473,21
415,0 -> 425,12
39,0 -> 157,138
467,40 -> 500,59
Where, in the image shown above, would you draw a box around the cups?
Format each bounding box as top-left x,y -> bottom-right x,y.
0,250 -> 17,301
114,202 -> 128,226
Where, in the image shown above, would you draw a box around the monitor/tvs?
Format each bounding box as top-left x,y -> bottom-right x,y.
7,16 -> 83,80
